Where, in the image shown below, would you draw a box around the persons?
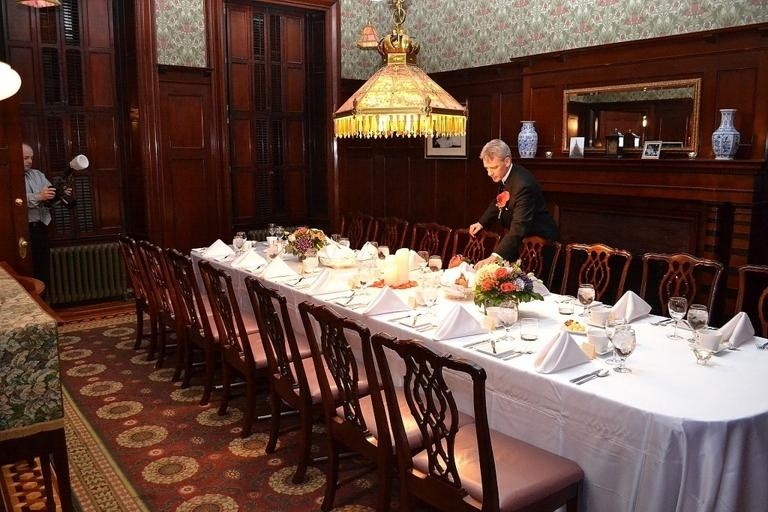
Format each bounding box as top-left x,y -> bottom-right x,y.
468,138 -> 564,294
22,142 -> 73,302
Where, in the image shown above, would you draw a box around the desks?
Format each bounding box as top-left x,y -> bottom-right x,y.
1,263 -> 75,511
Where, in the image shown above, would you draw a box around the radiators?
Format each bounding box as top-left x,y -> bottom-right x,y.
49,241 -> 135,307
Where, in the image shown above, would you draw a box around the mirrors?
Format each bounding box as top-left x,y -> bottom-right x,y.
560,74 -> 703,158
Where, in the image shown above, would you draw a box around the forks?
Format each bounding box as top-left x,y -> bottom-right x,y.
500,349 -> 534,361
757,341 -> 768,350
325,292 -> 354,301
650,316 -> 674,327
389,314 -> 411,322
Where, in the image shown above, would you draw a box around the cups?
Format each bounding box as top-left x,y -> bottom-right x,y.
587,328 -> 611,351
558,294 -> 575,315
266,237 -> 276,248
698,328 -> 723,350
302,259 -> 314,273
246,241 -> 252,249
589,306 -> 610,324
519,316 -> 539,340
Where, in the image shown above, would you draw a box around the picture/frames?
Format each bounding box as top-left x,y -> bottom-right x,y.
641,140 -> 662,160
423,102 -> 470,160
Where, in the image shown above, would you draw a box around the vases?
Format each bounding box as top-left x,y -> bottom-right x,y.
518,118 -> 538,160
484,302 -> 518,327
710,108 -> 741,161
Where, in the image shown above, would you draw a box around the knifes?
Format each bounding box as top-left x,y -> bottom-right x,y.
569,369 -> 599,383
412,316 -> 417,327
294,276 -> 303,285
345,297 -> 354,304
490,340 -> 497,354
464,338 -> 490,347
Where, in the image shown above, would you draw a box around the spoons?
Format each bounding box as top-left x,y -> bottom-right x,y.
577,368 -> 609,386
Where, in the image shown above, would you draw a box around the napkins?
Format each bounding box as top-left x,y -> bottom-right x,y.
204,239 -> 235,260
533,330 -> 592,375
363,285 -> 409,316
607,290 -> 653,323
357,242 -> 377,261
308,267 -> 349,297
432,305 -> 488,341
408,250 -> 427,270
715,311 -> 754,348
522,276 -> 552,301
263,256 -> 296,279
232,248 -> 267,268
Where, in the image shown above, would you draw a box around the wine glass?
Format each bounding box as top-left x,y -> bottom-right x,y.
266,223 -> 285,243
685,304 -> 709,344
417,250 -> 444,318
578,284 -> 596,317
497,301 -> 519,339
605,317 -> 636,374
332,234 -> 389,274
667,296 -> 688,341
233,230 -> 248,254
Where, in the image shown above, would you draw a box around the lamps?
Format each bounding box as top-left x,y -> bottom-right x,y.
357,0 -> 381,50
330,1 -> 468,140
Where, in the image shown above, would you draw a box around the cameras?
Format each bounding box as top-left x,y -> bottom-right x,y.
43,154 -> 89,210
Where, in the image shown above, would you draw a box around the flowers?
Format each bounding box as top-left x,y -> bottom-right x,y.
286,228 -> 326,258
472,260 -> 539,306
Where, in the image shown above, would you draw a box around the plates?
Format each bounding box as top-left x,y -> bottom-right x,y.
440,274 -> 475,288
594,348 -> 612,355
560,325 -> 589,334
399,318 -> 429,328
477,341 -> 516,355
286,280 -> 306,287
702,346 -> 726,354
335,296 -> 362,307
215,257 -> 232,262
585,319 -> 606,328
574,299 -> 601,307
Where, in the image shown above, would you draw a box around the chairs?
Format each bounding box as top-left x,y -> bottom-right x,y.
411,220 -> 451,273
242,272 -> 383,509
372,216 -> 408,257
196,259 -> 323,438
370,328 -> 587,511
451,230 -> 501,278
559,241 -> 632,307
168,246 -> 262,407
509,234 -> 560,292
141,240 -> 224,381
186,234 -> 765,508
298,301 -> 477,507
122,236 -> 196,362
729,264 -> 768,337
337,210 -> 373,250
639,249 -> 724,327
